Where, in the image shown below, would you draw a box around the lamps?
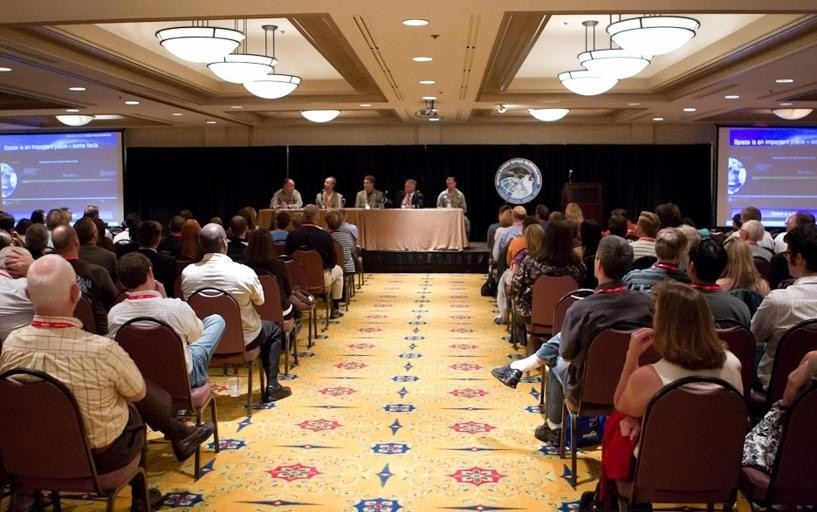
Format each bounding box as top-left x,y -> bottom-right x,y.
299,109 -> 340,126
558,65 -> 620,97
206,18 -> 280,86
578,19 -> 655,81
606,12 -> 702,55
51,107 -> 96,128
154,20 -> 246,65
240,20 -> 302,102
768,102 -> 816,120
497,102 -> 508,114
526,108 -> 572,122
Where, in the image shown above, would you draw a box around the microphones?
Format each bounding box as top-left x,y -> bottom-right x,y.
321,188 -> 326,194
568,169 -> 573,178
382,190 -> 389,198
416,190 -> 420,194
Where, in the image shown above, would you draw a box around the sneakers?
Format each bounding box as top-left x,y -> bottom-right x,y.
494,317 -> 509,325
130,487 -> 162,512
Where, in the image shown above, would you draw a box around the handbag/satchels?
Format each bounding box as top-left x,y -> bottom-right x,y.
290,286 -> 316,311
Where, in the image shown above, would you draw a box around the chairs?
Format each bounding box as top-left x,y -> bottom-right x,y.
556,328 -> 639,488
183,286 -> 267,418
113,313 -> 220,481
746,377 -> 817,509
746,315 -> 817,413
588,374 -> 748,507
536,257 -> 799,408
1,228 -> 363,379
1,366 -> 157,512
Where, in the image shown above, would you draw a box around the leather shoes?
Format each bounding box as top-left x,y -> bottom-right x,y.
173,420 -> 214,461
330,309 -> 344,319
491,363 -> 522,389
535,422 -> 561,446
266,387 -> 291,402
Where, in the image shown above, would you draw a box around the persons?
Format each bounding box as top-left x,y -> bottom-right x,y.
305,176 -> 346,209
267,177 -> 303,210
352,175 -> 385,210
479,201 -> 816,511
394,177 -> 424,209
0,203 -> 362,499
434,174 -> 471,245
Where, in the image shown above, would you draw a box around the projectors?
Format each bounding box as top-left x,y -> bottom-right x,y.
415,110 -> 440,120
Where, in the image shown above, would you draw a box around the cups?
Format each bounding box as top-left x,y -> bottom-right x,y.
227,377 -> 241,398
